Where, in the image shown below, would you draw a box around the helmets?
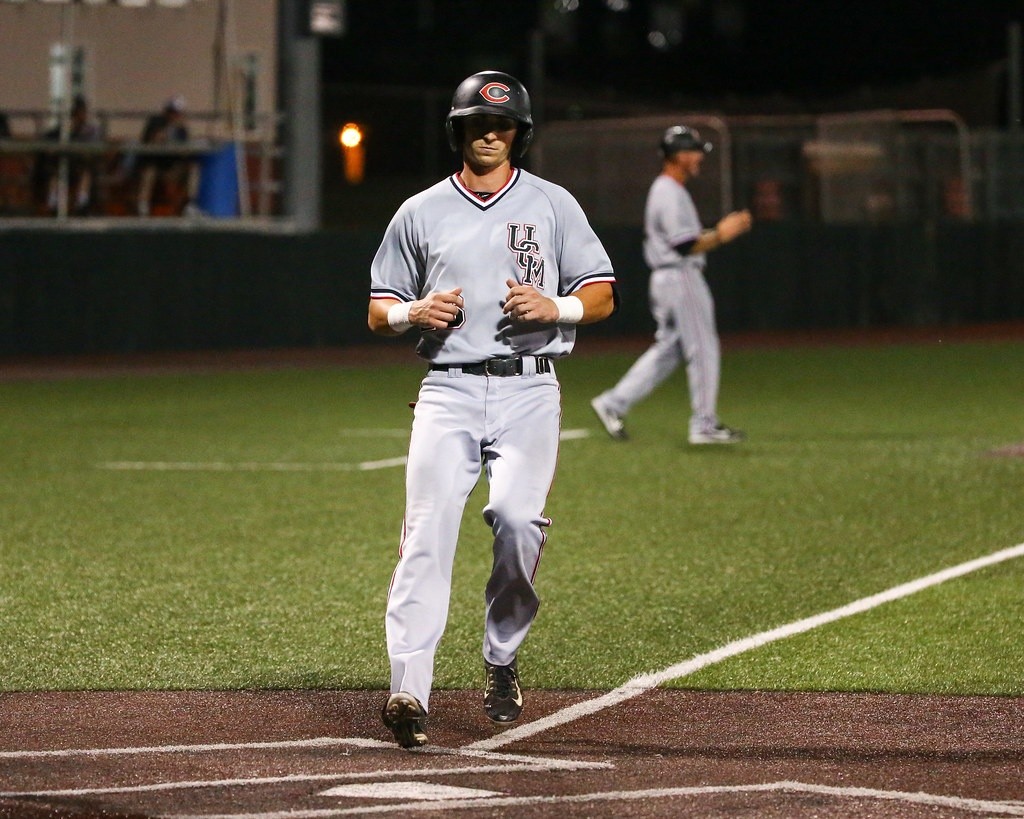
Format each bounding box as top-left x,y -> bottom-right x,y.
446,70 -> 533,158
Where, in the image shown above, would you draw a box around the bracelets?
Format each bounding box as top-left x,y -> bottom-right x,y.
548,295 -> 584,324
388,300 -> 414,332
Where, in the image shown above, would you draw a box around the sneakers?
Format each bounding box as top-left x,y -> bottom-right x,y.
381,693 -> 428,747
689,424 -> 745,443
591,396 -> 627,441
483,656 -> 524,724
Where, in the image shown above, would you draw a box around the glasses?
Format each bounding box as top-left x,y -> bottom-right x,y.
462,117 -> 516,131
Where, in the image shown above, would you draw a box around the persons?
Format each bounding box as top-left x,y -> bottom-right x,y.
368,72 -> 617,747
38,93 -> 97,217
591,127 -> 751,444
132,96 -> 189,217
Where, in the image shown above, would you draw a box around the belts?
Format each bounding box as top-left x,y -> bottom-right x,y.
429,356 -> 550,376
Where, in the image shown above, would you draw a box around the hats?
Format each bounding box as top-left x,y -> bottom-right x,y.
662,125 -> 714,152
